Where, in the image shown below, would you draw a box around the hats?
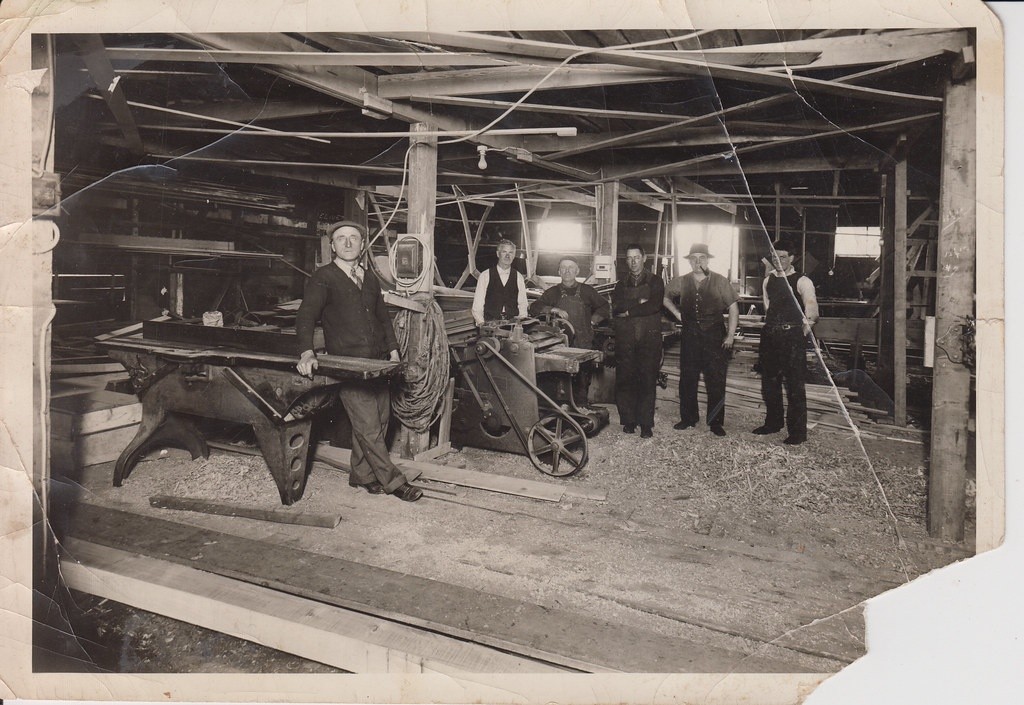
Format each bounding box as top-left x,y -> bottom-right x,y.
325,220 -> 367,239
682,244 -> 714,260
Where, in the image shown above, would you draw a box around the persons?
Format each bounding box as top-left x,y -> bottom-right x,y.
751,239 -> 820,446
295,220 -> 424,503
530,255 -> 611,413
662,242 -> 740,436
471,238 -> 528,327
612,242 -> 665,438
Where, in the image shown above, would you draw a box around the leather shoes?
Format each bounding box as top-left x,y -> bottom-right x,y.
349,480 -> 384,494
394,481 -> 423,502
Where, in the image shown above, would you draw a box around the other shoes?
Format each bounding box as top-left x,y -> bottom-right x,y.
752,424 -> 785,436
640,427 -> 653,438
623,425 -> 636,434
783,435 -> 806,445
674,421 -> 697,430
710,424 -> 726,436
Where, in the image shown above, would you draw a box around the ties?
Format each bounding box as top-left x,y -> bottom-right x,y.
350,269 -> 362,290
